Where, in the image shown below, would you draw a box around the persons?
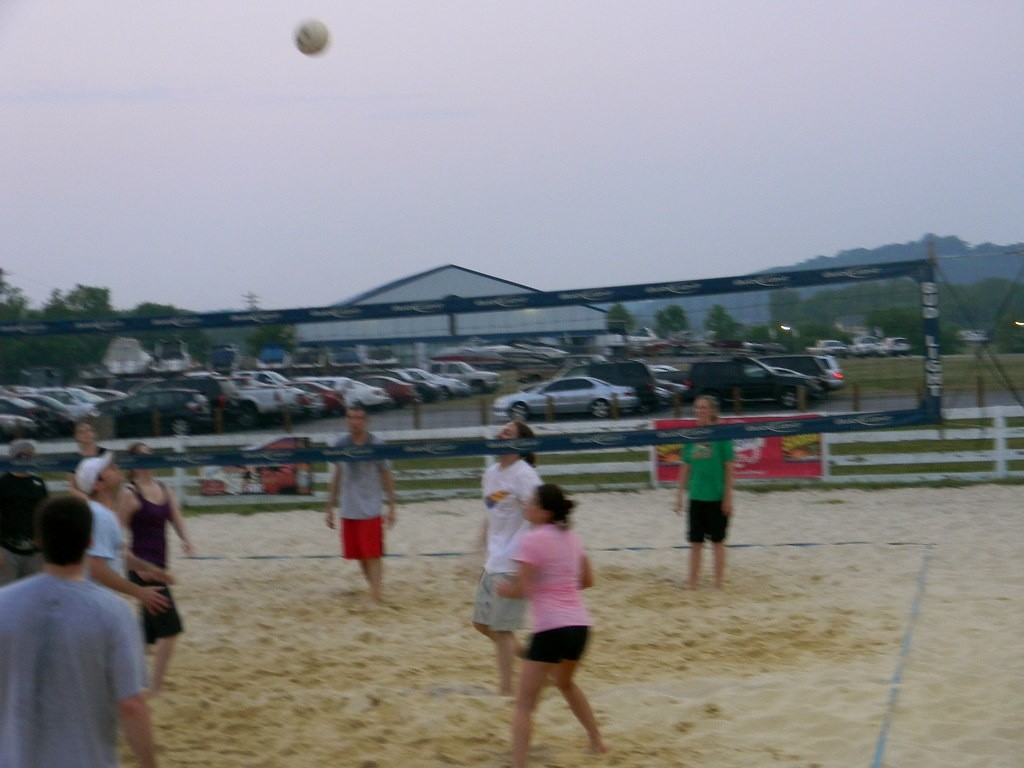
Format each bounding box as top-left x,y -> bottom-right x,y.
673,395 -> 735,587
0,438 -> 49,587
494,483 -> 608,768
325,403 -> 397,595
470,421 -> 545,694
76,451 -> 179,616
117,442 -> 193,691
66,422 -> 107,489
0,494 -> 157,768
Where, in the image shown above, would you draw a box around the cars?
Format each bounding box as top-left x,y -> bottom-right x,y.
85,384 -> 213,436
849,336 -> 887,357
0,369 -> 470,441
492,376 -> 639,422
427,335 -> 845,407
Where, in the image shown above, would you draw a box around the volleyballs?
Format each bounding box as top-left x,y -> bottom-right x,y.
291,17 -> 331,56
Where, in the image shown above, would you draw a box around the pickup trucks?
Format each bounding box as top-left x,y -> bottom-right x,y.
806,339 -> 847,358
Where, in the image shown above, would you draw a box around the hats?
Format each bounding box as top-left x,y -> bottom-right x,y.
74,449 -> 114,494
9,437 -> 35,458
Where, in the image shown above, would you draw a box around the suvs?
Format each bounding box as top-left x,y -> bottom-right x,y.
557,359 -> 658,415
886,337 -> 911,355
688,356 -> 827,408
429,361 -> 501,393
